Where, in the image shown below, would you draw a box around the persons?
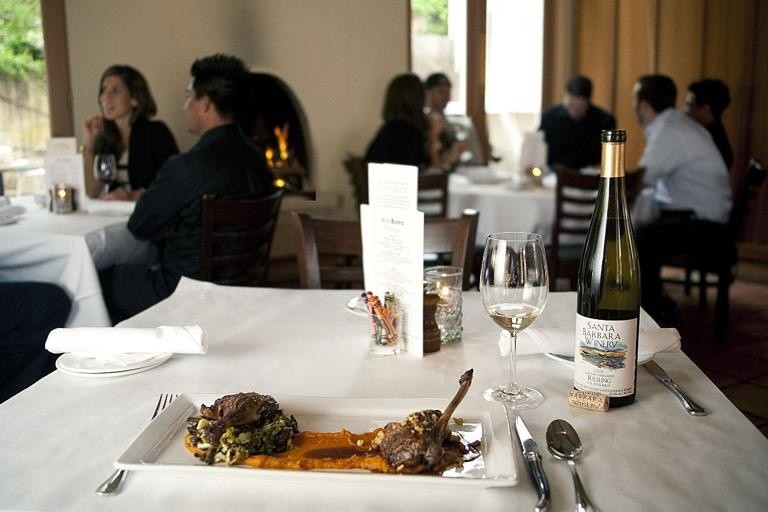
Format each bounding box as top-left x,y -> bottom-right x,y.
80,65 -> 180,202
540,75 -> 616,173
0,281 -> 72,388
684,78 -> 735,171
364,74 -> 430,176
98,54 -> 276,326
424,74 -> 472,170
631,74 -> 733,339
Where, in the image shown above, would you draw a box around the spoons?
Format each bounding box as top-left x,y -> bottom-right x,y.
545,420 -> 597,512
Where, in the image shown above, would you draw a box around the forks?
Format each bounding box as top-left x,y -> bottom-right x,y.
95,394 -> 180,498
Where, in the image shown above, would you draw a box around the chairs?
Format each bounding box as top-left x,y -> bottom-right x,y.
200,188 -> 289,285
417,168 -> 452,272
538,162 -> 649,293
645,156 -> 765,334
289,209 -> 480,292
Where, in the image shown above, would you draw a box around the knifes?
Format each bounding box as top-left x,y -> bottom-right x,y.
643,360 -> 712,416
515,416 -> 548,512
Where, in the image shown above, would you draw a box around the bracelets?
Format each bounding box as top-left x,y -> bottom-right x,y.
79,145 -> 96,157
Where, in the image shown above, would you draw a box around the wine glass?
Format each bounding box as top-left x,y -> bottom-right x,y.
480,232 -> 551,410
93,153 -> 116,202
454,115 -> 473,162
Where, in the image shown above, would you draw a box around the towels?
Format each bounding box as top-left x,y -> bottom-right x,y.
498,327 -> 683,355
45,324 -> 211,355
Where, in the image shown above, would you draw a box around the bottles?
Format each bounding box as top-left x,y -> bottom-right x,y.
569,126 -> 644,410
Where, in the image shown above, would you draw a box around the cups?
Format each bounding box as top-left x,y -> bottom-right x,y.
367,307 -> 404,355
423,265 -> 465,343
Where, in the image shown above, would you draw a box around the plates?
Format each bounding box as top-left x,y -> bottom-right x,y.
544,350 -> 655,366
55,352 -> 175,378
114,395 -> 517,489
